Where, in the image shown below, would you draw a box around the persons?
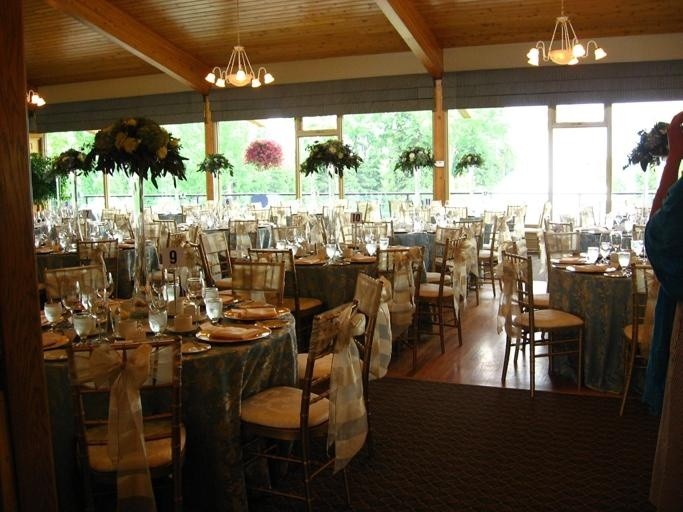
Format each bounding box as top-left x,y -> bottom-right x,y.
642,112 -> 683,512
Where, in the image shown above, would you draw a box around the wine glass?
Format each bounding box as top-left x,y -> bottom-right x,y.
391,208 -> 463,233
587,230 -> 648,279
612,208 -> 650,226
33,207 -> 124,252
43,269 -> 222,341
186,203 -> 389,266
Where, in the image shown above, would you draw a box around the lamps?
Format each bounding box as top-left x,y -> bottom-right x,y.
25,85 -> 47,110
528,1 -> 608,69
203,0 -> 275,90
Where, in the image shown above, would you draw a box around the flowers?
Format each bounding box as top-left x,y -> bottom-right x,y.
392,140 -> 435,178
620,119 -> 683,174
84,117 -> 187,187
452,151 -> 488,177
49,148 -> 99,180
301,137 -> 364,179
198,149 -> 233,181
244,139 -> 283,170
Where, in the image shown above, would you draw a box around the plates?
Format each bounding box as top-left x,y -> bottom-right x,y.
39,310 -> 68,361
175,294 -> 292,354
